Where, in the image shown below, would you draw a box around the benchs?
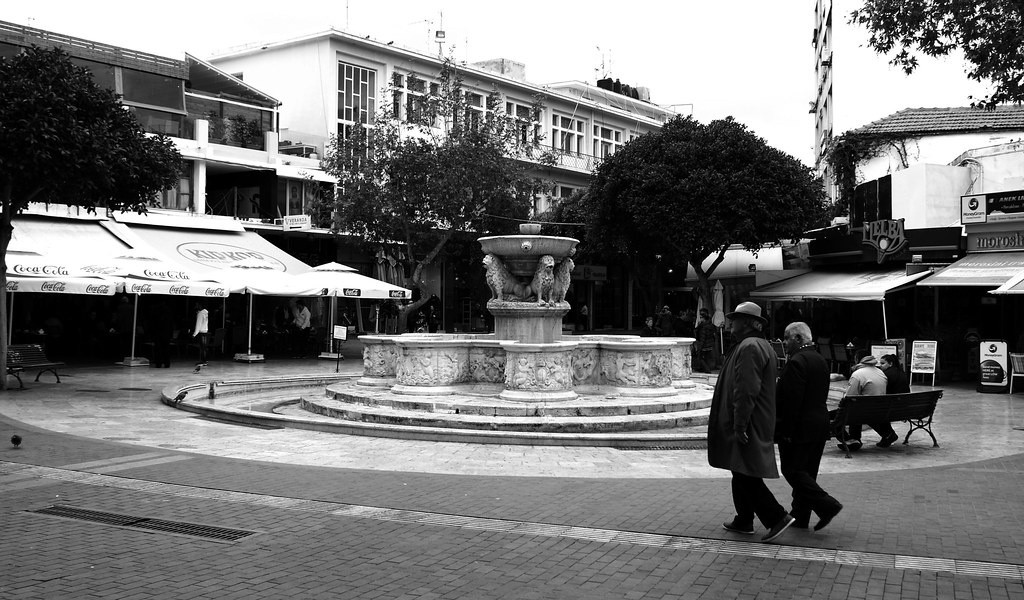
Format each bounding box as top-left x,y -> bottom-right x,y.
6,344 -> 65,388
827,388 -> 944,459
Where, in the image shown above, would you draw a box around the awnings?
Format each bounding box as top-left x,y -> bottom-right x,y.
749,269 -> 936,301
0,217 -> 313,297
916,252 -> 1024,286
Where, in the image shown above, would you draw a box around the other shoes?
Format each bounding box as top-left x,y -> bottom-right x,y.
722,522 -> 755,534
837,439 -> 862,451
761,515 -> 796,542
196,360 -> 208,366
791,523 -> 808,528
814,501 -> 842,531
876,431 -> 898,448
291,356 -> 309,359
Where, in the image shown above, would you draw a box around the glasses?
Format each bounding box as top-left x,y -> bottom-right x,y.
700,317 -> 703,319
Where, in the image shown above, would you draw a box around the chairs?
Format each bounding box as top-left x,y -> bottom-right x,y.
1008,351 -> 1024,394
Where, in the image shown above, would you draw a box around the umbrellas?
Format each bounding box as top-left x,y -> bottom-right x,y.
372,246 -> 406,334
296,262 -> 412,358
987,270 -> 1024,294
715,280 -> 724,355
695,295 -> 704,328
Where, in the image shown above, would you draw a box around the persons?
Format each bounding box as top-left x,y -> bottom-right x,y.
830,350 -> 888,453
292,301 -> 311,358
870,353 -> 910,447
775,321 -> 841,530
423,306 -> 438,332
277,301 -> 294,325
640,306 -> 713,372
707,303 -> 795,544
193,306 -> 209,366
153,325 -> 173,367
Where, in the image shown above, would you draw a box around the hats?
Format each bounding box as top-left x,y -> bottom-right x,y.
296,299 -> 305,304
724,302 -> 768,327
